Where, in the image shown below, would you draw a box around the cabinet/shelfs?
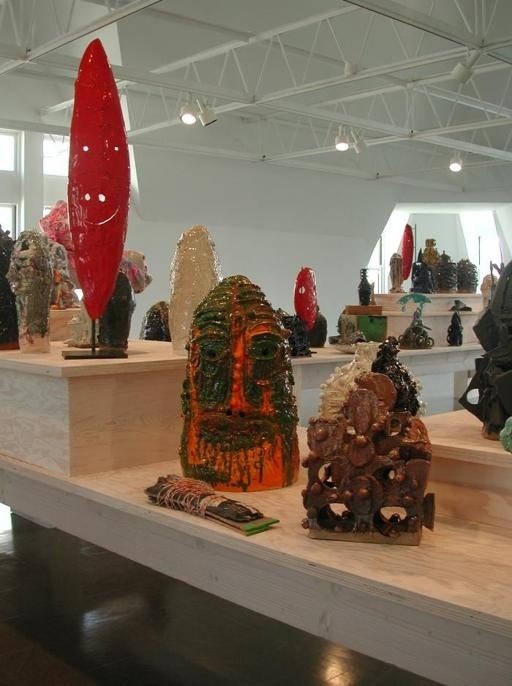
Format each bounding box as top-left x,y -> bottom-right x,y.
0,344 -> 512,685
373,292 -> 492,346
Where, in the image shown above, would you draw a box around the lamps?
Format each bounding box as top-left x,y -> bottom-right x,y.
177,92 -> 221,130
447,150 -> 466,176
449,48 -> 485,85
333,124 -> 370,157
